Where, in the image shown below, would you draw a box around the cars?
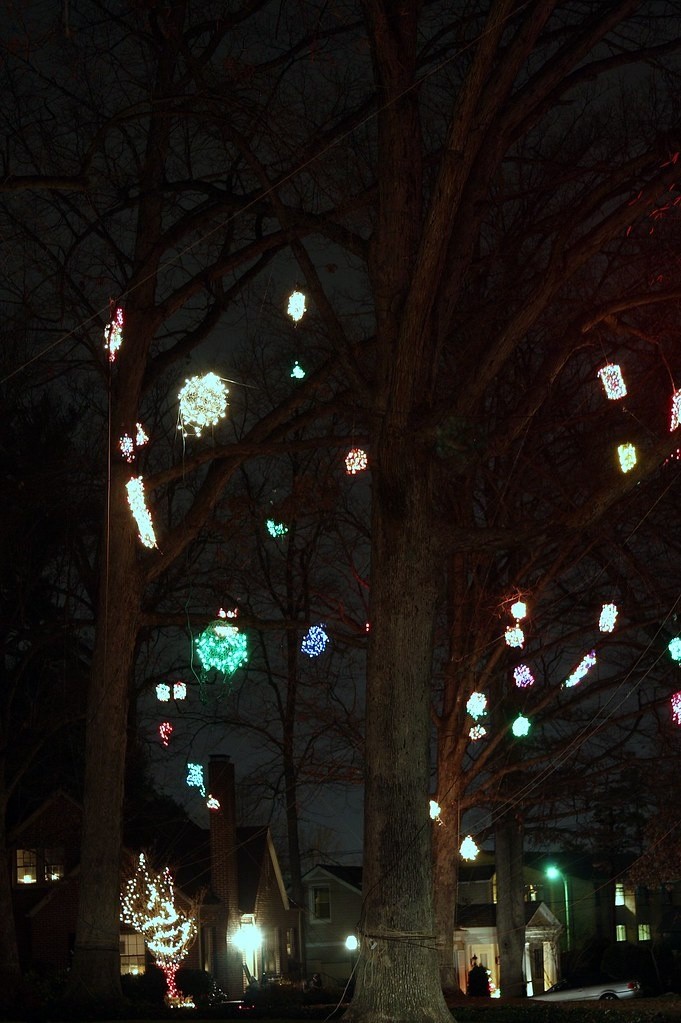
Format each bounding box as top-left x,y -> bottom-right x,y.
525,975 -> 645,1003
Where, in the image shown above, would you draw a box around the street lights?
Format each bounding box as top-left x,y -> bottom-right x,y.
545,866 -> 571,952
